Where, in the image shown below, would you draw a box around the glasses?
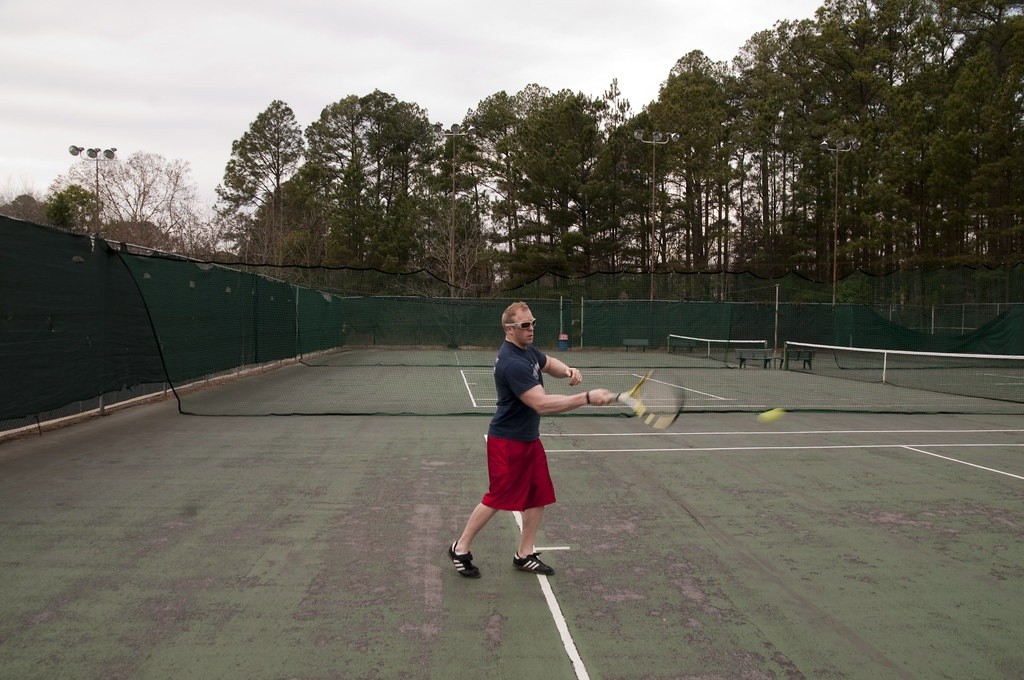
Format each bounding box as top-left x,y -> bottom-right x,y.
503,318 -> 536,329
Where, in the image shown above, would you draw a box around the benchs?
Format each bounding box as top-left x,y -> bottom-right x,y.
669,340 -> 697,353
734,349 -> 778,369
778,349 -> 816,370
622,339 -> 651,352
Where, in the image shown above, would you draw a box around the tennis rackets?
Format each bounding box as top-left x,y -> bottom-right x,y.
606,365 -> 686,429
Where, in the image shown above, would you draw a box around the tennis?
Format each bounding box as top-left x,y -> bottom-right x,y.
756,407 -> 784,426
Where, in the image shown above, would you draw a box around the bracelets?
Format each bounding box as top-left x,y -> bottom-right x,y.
585,391 -> 591,404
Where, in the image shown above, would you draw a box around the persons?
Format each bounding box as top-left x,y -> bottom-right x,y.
448,302 -> 611,579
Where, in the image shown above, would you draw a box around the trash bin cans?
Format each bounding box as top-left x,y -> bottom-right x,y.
559,334 -> 569,349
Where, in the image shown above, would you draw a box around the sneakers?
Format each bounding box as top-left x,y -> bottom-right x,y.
447,540 -> 482,579
512,550 -> 555,576
